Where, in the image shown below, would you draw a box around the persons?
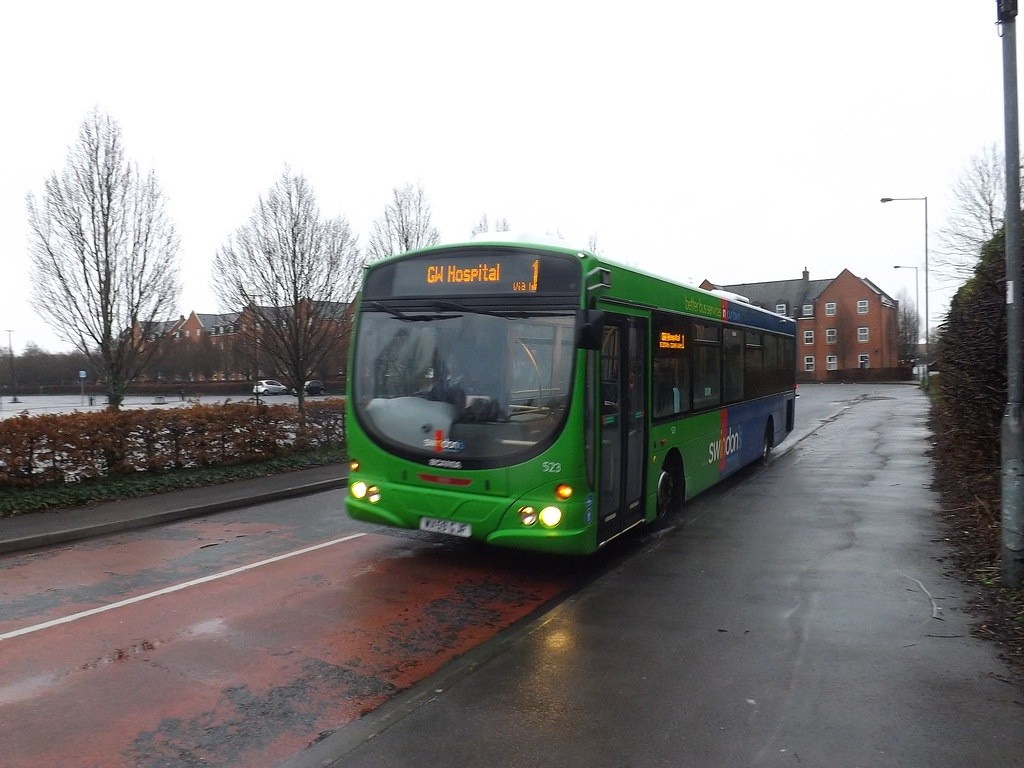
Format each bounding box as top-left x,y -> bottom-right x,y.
431,352 -> 474,405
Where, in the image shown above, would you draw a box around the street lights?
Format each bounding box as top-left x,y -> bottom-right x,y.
881,197 -> 929,379
892,266 -> 919,343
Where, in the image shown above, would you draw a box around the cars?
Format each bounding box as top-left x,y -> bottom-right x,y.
291,380 -> 326,397
252,380 -> 288,396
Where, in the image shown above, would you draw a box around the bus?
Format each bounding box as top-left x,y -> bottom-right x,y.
343,241 -> 798,555
